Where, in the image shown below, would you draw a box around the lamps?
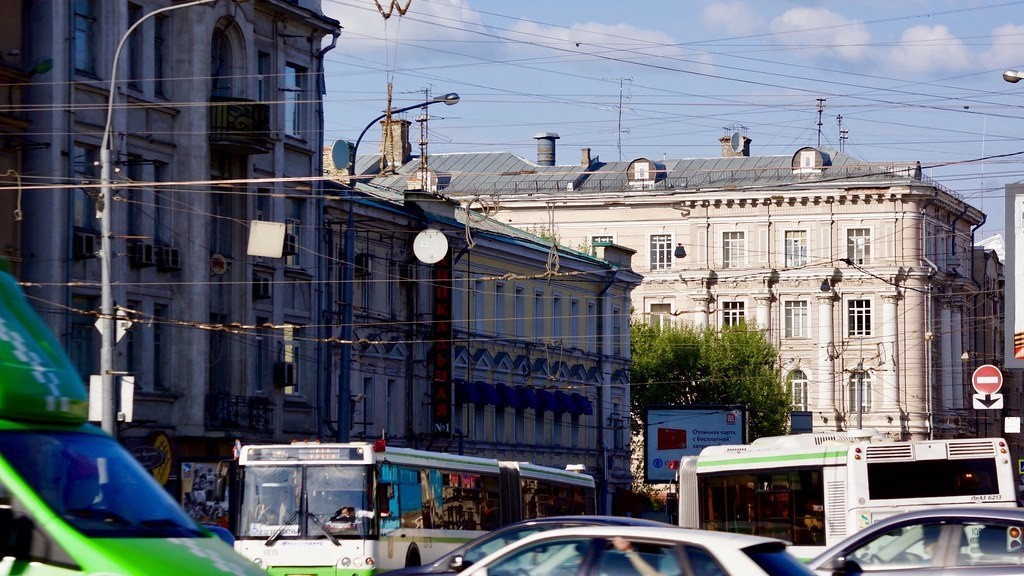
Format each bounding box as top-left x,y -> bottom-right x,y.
412,227 -> 450,263
819,275 -> 834,292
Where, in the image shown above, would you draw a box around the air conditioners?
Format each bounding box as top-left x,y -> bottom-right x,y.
162,246 -> 180,267
283,234 -> 299,252
75,233 -> 95,254
134,242 -> 154,264
281,362 -> 296,385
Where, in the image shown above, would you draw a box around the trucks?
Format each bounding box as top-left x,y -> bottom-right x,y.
0,271 -> 274,576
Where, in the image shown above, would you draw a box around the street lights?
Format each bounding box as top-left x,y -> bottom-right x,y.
337,92 -> 461,443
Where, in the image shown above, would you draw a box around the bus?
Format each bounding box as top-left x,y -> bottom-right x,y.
665,430 -> 1018,564
235,438 -> 596,576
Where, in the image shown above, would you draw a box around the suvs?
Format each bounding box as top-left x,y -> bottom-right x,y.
371,507 -> 1023,575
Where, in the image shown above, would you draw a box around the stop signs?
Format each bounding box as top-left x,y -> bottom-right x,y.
972,364 -> 1003,394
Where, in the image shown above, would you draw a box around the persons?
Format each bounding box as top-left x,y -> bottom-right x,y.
337,505 -> 393,519
50,456 -> 98,518
922,529 -> 939,563
185,465 -> 226,519
